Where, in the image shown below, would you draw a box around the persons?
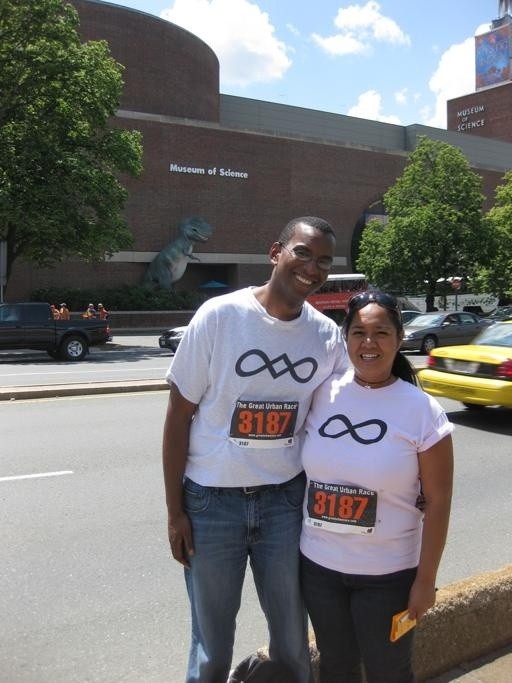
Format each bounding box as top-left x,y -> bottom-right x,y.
85,303 -> 96,320
299,286 -> 456,681
51,304 -> 59,319
97,302 -> 110,339
59,302 -> 70,320
162,214 -> 361,682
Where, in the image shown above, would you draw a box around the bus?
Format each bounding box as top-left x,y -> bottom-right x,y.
396,276 -> 512,317
304,273 -> 369,324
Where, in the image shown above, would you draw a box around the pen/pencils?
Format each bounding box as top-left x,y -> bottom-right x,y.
400,588 -> 439,623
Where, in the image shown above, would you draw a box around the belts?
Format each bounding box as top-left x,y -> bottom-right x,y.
222,484 -> 278,493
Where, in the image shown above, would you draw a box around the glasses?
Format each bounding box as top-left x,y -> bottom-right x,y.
283,244 -> 332,271
349,292 -> 398,309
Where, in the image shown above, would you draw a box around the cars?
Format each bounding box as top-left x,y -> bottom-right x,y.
159,325 -> 189,353
398,305 -> 512,412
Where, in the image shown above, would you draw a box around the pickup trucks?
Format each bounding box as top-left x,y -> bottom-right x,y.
0,301 -> 111,362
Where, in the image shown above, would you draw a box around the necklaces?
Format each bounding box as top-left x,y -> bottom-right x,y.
354,373 -> 391,389
